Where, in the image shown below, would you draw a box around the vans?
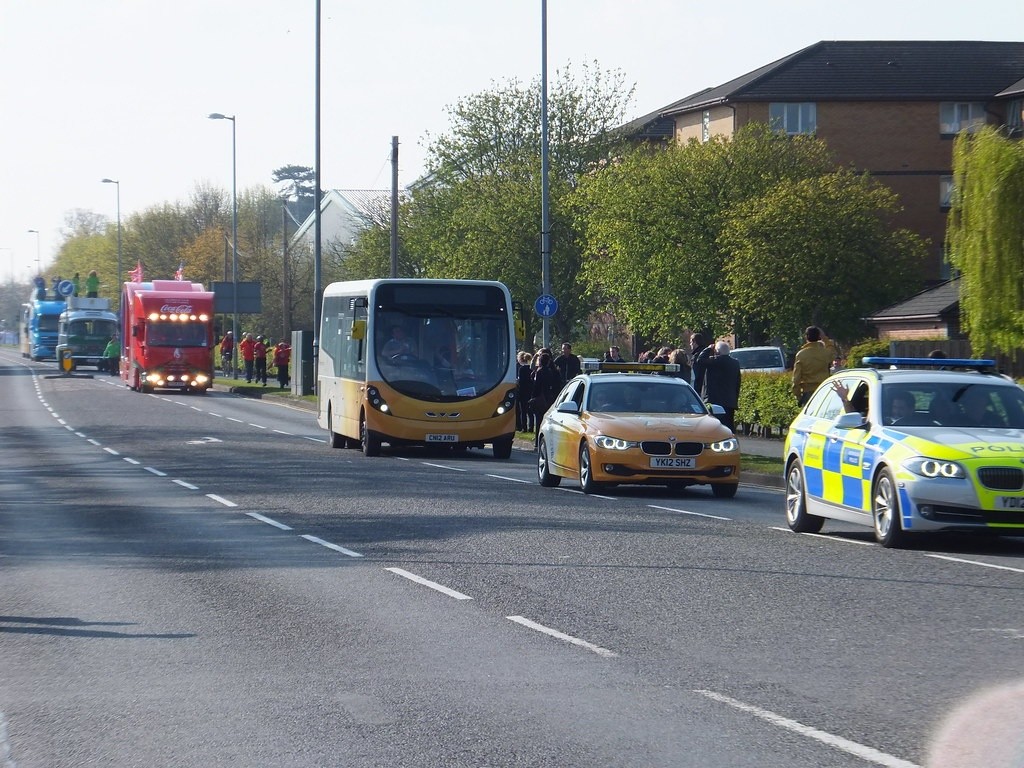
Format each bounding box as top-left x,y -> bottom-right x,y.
729,346 -> 785,373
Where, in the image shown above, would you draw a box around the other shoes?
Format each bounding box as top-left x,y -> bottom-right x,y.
246,379 -> 288,389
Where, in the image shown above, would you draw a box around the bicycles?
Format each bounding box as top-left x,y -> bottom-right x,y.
219,351 -> 233,378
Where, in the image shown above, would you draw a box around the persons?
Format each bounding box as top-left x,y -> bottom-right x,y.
220,331 -> 234,360
930,394 -> 956,427
637,347 -> 691,385
173,326 -> 187,340
32,273 -> 45,300
829,380 -> 916,425
690,332 -> 741,434
517,343 -> 582,434
102,333 -> 120,376
152,326 -> 167,342
594,391 -> 619,412
951,389 -> 1008,428
791,325 -> 840,408
928,351 -> 950,371
51,271 -> 100,300
273,338 -> 291,388
601,347 -> 629,373
240,333 -> 272,386
382,326 -> 418,365
830,357 -> 845,375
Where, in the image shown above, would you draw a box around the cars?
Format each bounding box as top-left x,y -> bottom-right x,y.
538,362 -> 741,498
783,357 -> 1024,547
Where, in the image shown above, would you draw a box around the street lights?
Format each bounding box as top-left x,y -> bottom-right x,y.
208,114 -> 238,381
102,179 -> 123,358
28,230 -> 41,276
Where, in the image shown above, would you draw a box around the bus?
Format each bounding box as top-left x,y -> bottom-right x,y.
317,279 -> 527,458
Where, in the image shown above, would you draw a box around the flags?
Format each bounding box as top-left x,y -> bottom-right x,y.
128,264 -> 143,283
174,263 -> 184,280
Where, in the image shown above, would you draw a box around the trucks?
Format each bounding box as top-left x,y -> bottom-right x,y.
56,297 -> 119,374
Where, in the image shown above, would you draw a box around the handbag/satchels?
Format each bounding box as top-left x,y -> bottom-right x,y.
528,393 -> 536,414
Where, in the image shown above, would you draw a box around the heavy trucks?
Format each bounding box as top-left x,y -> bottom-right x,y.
18,300 -> 68,361
119,280 -> 215,393
